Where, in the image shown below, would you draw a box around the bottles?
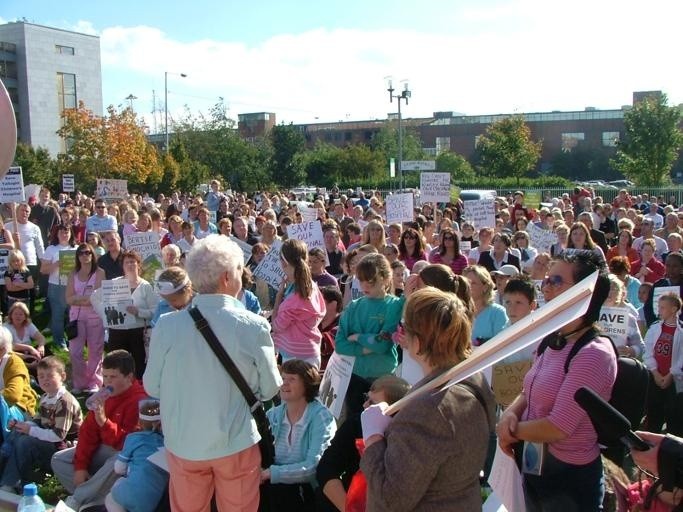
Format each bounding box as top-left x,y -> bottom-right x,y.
17,484 -> 46,512
86,385 -> 113,412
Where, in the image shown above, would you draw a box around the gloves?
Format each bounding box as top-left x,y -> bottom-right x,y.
360,401 -> 393,441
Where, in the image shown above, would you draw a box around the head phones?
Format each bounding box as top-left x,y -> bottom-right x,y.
548,321 -> 596,350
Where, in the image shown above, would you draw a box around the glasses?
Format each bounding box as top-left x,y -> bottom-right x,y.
77,250 -> 92,254
541,274 -> 576,290
444,237 -> 455,241
403,235 -> 415,240
95,206 -> 105,209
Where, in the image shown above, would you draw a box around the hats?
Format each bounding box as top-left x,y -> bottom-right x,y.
489,264 -> 520,276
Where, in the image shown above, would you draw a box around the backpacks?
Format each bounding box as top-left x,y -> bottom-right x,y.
538,327 -> 650,468
185,304 -> 277,469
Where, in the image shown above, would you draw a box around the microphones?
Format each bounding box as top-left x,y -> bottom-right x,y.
573,386 -> 649,451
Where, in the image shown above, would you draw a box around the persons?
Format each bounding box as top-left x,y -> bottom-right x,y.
237,268 -> 261,313
66,243 -> 106,396
0,216 -> 14,316
1,327 -> 40,450
260,221 -> 282,244
158,244 -> 181,266
52,350 -> 148,512
274,240 -> 327,372
315,375 -> 412,511
244,244 -> 267,271
27,186 -> 124,245
103,250 -> 159,382
151,268 -> 197,327
218,216 -> 233,237
232,186 -> 283,220
5,204 -> 45,316
3,301 -> 56,386
501,278 -> 536,329
121,187 -> 205,217
122,210 -> 140,248
317,252 -> 403,417
627,430 -> 682,490
176,222 -> 198,257
234,219 -> 259,245
86,199 -> 119,254
136,214 -> 152,233
97,229 -> 127,280
283,184 -> 682,290
644,292 -> 683,429
592,275 -> 644,359
192,208 -> 218,238
361,288 -> 496,511
40,224 -> 81,355
464,265 -> 510,348
28,188 -> 61,298
85,233 -> 105,261
215,199 -> 233,222
260,361 -> 337,511
398,264 -> 469,386
201,180 -> 230,210
143,234 -> 282,511
497,249 -> 618,512
160,215 -> 184,248
1,357 -> 84,486
